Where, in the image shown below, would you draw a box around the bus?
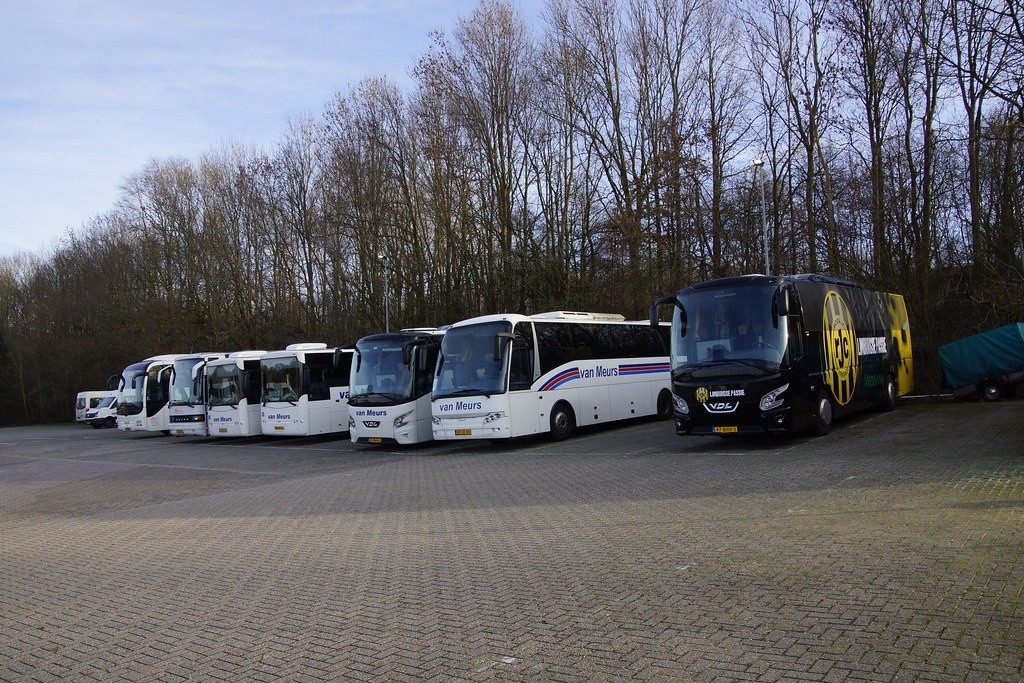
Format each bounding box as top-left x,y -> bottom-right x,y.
204,347 -> 268,438
257,339 -> 357,436
106,353 -> 201,438
429,309 -> 691,442
158,352 -> 234,439
331,327 -> 485,449
648,271 -> 917,438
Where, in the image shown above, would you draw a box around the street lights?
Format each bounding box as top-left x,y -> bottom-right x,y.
378,256 -> 389,334
752,159 -> 771,276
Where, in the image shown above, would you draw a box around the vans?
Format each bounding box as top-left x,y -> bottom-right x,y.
75,391 -> 131,427
91,389 -> 140,428
